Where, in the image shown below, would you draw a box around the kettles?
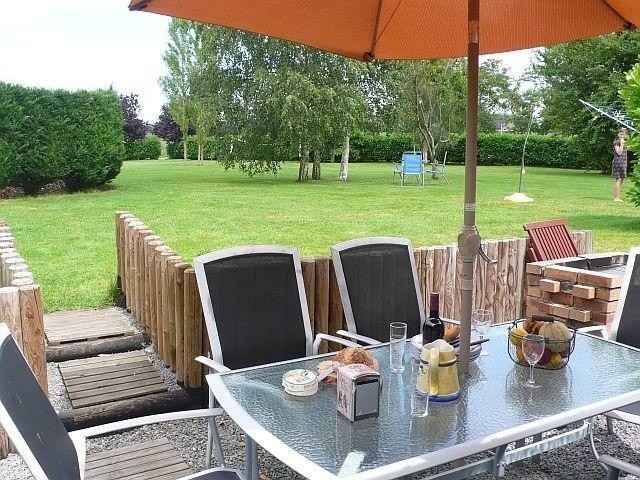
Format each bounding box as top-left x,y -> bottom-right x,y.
417,341 -> 461,400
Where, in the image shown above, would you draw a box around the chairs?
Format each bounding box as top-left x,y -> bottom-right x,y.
391,151 -> 451,186
328,235 -> 474,349
191,242 -> 369,471
0,321 -> 246,480
574,245 -> 640,438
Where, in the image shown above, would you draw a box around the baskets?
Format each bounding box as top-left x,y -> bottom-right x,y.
507,316 -> 577,370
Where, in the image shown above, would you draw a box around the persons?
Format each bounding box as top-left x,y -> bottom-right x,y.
611,127 -> 628,202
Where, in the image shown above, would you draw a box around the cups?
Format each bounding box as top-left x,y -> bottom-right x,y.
389,323 -> 430,419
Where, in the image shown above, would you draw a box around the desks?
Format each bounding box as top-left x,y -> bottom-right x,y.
202,314 -> 640,478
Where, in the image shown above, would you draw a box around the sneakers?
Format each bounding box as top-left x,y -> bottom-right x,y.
614,199 -> 622,201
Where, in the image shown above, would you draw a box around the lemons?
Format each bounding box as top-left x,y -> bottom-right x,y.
510,327 -> 530,346
533,322 -> 544,334
523,318 -> 537,334
549,353 -> 563,368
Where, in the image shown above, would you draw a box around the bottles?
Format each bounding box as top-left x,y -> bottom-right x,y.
423,292 -> 444,344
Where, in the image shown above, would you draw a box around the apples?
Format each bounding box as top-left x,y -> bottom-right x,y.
528,341 -> 551,366
517,343 -> 534,364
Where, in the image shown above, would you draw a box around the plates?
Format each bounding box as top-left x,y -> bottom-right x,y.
410,332 -> 482,364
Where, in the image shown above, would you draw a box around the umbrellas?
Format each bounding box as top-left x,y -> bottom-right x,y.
128,1 -> 639,375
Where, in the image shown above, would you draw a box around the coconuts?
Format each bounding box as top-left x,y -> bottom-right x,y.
540,321 -> 570,352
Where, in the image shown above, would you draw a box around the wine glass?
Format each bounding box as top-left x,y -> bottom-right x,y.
522,335 -> 545,389
473,309 -> 492,356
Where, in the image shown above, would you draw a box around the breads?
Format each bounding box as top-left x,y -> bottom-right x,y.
443,324 -> 461,342
333,346 -> 374,375
